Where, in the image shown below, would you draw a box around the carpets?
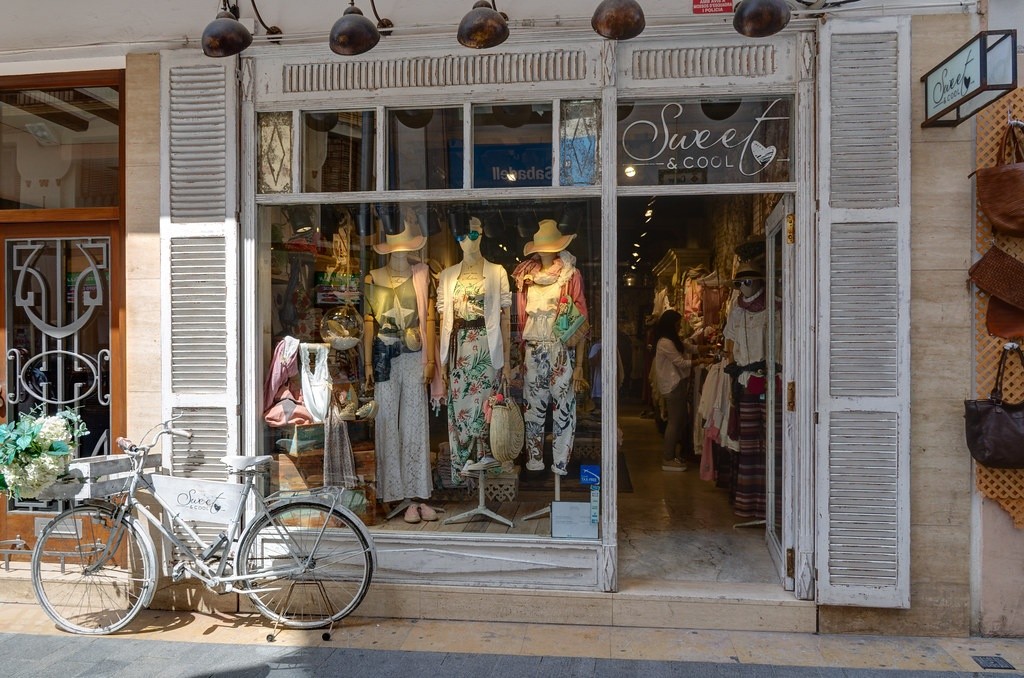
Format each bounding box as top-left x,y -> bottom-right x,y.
519,451 -> 634,493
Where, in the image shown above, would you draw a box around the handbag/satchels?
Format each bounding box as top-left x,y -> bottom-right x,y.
490,378 -> 524,461
404,328 -> 423,351
554,293 -> 589,347
961,121 -> 1024,470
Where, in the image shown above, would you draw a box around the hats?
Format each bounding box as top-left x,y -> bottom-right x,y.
372,220 -> 428,255
523,219 -> 577,256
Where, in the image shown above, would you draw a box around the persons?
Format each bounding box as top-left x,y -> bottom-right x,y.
434,217 -> 512,487
650,310 -> 713,472
363,223 -> 435,501
727,264 -> 771,519
512,219 -> 591,476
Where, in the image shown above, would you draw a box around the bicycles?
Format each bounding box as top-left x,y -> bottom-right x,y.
30,409 -> 380,642
268,438 -> 377,527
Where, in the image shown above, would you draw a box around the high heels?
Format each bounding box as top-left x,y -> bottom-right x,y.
662,458 -> 687,471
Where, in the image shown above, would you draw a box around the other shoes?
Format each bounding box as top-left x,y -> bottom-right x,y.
461,460 -> 480,478
356,400 -> 378,419
468,457 -> 502,470
403,504 -> 421,523
418,504 -> 438,520
339,402 -> 356,420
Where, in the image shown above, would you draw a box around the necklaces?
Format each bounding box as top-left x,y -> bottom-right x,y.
742,286 -> 764,303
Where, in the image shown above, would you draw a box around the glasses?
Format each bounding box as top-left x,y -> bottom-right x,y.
456,231 -> 482,241
734,279 -> 755,288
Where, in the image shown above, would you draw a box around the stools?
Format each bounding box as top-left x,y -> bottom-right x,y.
484,465 -> 519,502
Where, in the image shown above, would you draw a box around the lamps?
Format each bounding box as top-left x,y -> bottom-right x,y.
591,0 -> 645,40
202,0 -> 283,59
456,0 -> 510,49
322,201 -> 585,237
329,0 -> 394,56
732,0 -> 791,38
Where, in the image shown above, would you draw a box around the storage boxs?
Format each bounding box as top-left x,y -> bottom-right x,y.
272,419 -> 377,527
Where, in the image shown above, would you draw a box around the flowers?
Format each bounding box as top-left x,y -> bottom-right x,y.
0,399 -> 90,502
